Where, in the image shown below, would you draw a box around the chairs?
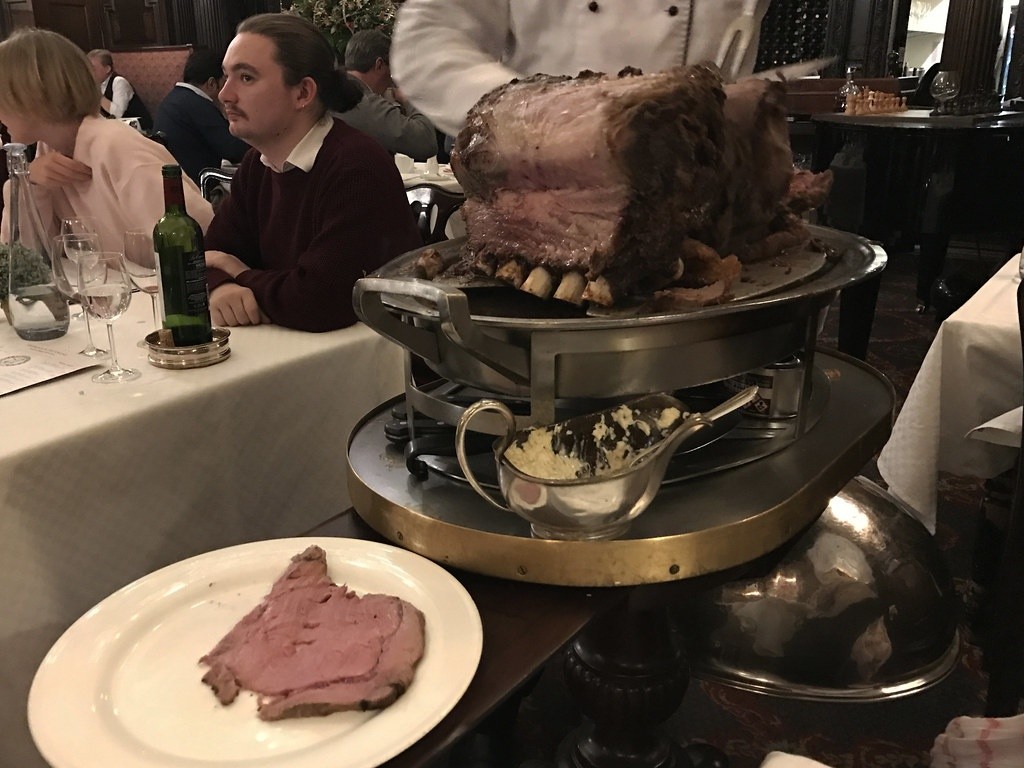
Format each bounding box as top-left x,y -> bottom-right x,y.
403,182 -> 467,249
113,46 -> 196,131
197,165 -> 233,216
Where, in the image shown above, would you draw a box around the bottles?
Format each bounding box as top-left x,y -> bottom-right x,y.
6,143 -> 70,340
835,67 -> 861,111
153,163 -> 214,348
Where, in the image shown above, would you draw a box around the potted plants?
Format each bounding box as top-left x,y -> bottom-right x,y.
0,242 -> 53,327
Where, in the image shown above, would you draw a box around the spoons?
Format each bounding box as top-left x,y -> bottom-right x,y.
610,385 -> 760,472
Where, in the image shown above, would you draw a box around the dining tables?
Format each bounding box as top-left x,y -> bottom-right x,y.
813,108 -> 1023,316
0,254 -> 410,768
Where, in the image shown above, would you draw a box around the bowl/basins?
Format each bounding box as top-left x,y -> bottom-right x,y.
458,390 -> 713,541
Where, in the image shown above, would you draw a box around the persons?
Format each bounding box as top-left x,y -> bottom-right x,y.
323,29 -> 441,164
388,0 -> 780,156
0,27 -> 215,272
197,12 -> 430,331
87,48 -> 156,138
150,46 -> 249,192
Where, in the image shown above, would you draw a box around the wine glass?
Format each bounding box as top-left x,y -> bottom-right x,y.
931,70 -> 962,113
122,226 -> 163,350
77,251 -> 140,377
60,217 -> 99,321
54,234 -> 113,360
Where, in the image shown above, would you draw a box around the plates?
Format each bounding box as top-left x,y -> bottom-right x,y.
27,538 -> 482,767
